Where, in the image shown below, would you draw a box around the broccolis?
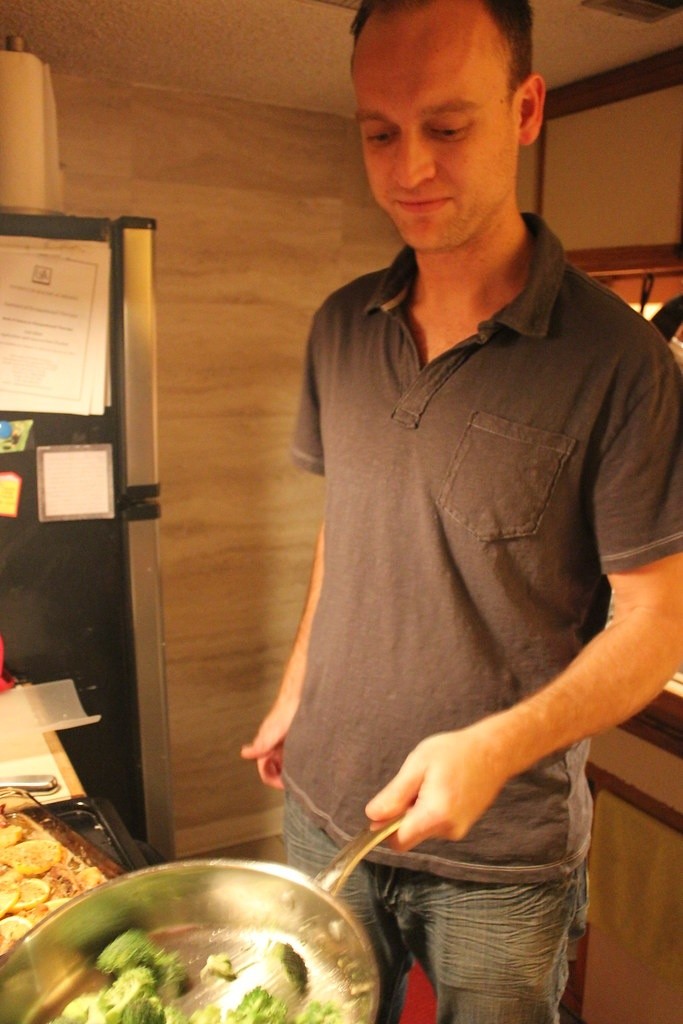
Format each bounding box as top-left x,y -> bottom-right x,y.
47,931 -> 352,1023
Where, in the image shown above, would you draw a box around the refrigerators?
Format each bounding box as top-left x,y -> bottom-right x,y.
0,215 -> 175,863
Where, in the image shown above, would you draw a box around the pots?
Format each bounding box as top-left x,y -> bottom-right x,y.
0,811 -> 410,1024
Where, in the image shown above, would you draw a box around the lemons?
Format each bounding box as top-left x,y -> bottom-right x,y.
0,826 -> 71,956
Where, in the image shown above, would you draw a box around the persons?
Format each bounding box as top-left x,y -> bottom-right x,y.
650,295 -> 683,375
241,1 -> 683,1024
